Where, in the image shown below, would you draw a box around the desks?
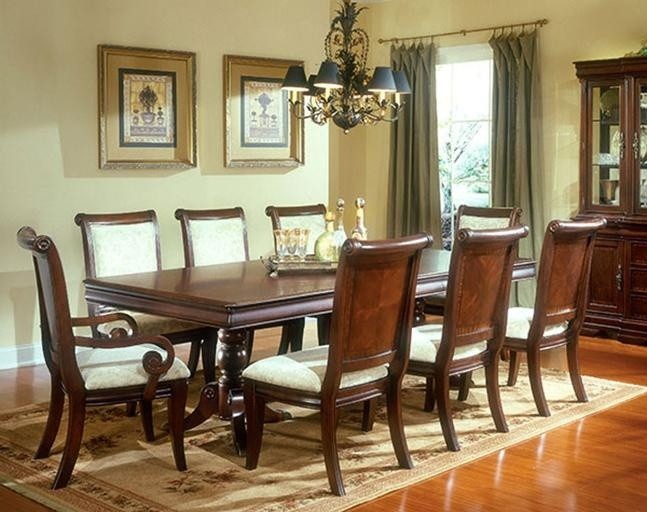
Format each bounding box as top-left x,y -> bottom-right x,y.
84,245 -> 535,407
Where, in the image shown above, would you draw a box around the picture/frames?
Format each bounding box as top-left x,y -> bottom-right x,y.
221,53 -> 305,168
96,42 -> 199,169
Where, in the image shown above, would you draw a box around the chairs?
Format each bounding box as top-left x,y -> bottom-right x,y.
16,226 -> 191,490
264,205 -> 333,255
243,231 -> 433,496
175,206 -> 292,357
449,203 -> 522,258
455,215 -> 607,416
74,209 -> 218,417
363,224 -> 530,451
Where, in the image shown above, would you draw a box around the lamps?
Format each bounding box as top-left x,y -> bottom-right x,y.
280,0 -> 410,133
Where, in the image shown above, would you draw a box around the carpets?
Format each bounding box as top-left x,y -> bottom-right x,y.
0,360 -> 647,510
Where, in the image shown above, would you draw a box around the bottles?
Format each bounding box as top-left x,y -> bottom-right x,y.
314,212 -> 334,261
351,197 -> 368,242
334,198 -> 347,260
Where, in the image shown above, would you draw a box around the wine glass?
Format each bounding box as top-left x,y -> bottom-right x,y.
297,229 -> 309,262
285,228 -> 300,261
270,229 -> 289,264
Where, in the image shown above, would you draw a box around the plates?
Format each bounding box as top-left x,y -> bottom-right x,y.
260,255 -> 335,278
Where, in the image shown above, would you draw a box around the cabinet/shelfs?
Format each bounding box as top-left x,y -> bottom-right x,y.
573,57 -> 646,346
